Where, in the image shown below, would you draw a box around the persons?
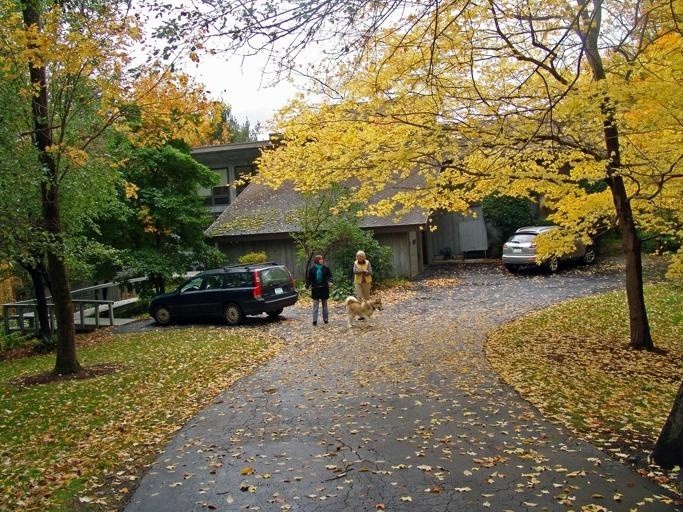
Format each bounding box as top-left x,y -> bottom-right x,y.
304,255 -> 334,325
351,251 -> 373,322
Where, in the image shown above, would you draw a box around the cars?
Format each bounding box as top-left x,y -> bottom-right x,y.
501,224 -> 598,273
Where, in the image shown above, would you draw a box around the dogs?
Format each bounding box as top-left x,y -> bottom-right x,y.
346,296 -> 385,328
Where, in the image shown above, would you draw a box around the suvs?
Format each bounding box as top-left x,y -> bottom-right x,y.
146,261 -> 298,325
